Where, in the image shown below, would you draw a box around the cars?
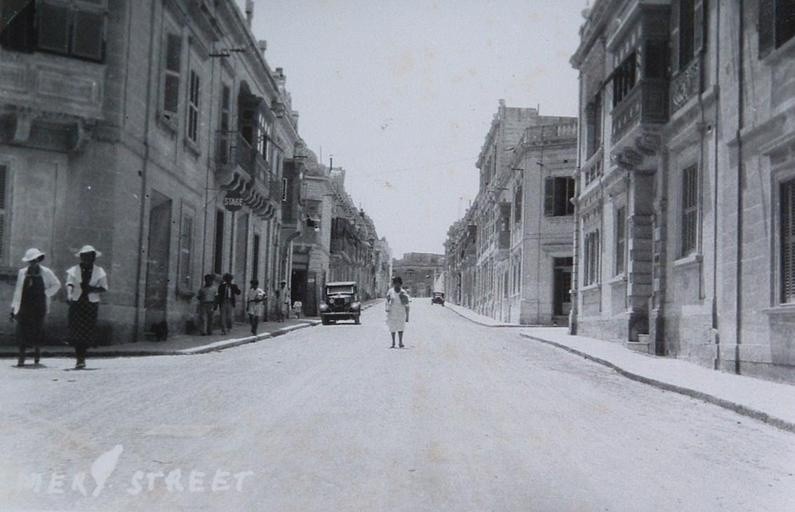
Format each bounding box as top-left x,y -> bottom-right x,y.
431,292 -> 445,306
319,282 -> 361,324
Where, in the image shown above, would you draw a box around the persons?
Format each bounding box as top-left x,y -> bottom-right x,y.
275,280 -> 288,322
66,244 -> 108,369
213,273 -> 241,335
246,279 -> 267,335
9,248 -> 62,368
293,298 -> 302,319
385,276 -> 410,348
196,273 -> 218,335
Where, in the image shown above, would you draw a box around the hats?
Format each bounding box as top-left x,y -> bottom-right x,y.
393,277 -> 402,284
75,245 -> 101,258
21,247 -> 44,262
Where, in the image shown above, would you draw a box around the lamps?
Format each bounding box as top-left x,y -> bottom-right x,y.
314,224 -> 319,232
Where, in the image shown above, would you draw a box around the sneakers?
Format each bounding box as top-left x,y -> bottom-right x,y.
224,328 -> 232,334
18,360 -> 39,366
251,328 -> 256,334
201,331 -> 212,335
391,344 -> 404,348
76,361 -> 85,367
